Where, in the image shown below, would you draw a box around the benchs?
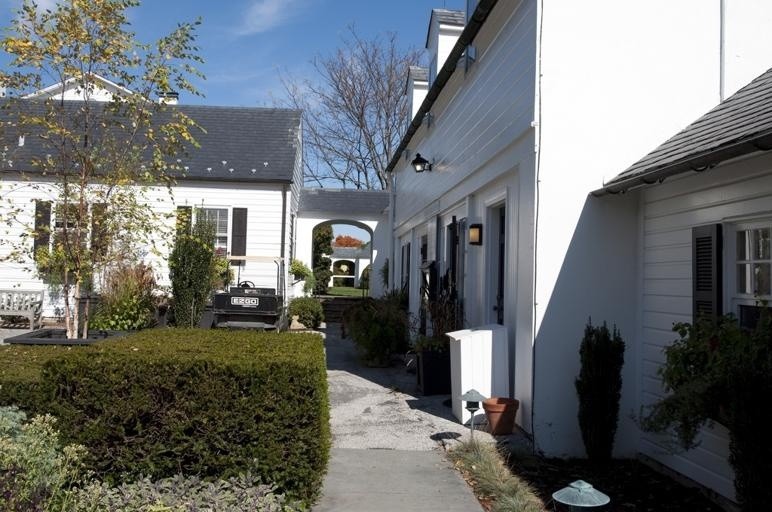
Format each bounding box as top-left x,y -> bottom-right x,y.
0,289 -> 44,332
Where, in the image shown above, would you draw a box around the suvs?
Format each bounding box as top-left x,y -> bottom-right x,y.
199,256 -> 290,333
